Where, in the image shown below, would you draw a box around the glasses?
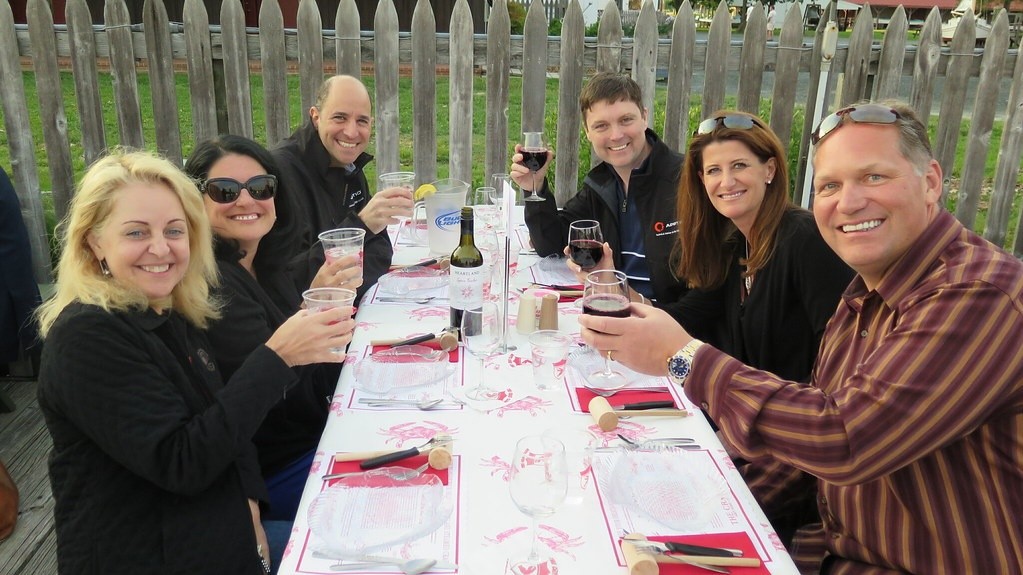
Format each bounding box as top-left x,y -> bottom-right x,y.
692,114 -> 766,138
808,104 -> 905,146
200,174 -> 277,204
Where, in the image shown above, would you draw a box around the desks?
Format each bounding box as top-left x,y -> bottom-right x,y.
276,205 -> 804,575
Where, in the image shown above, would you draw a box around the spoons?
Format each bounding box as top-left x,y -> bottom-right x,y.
369,398 -> 443,409
330,558 -> 437,575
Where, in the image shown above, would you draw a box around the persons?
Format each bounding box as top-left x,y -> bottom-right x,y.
511,72 -> 686,303
265,74 -> 416,323
18,145 -> 357,575
0,159 -> 42,363
178,134 -> 365,519
577,99 -> 1023,575
563,110 -> 832,429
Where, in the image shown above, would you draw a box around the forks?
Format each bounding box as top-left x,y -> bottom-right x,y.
376,351 -> 448,361
583,385 -> 668,397
321,462 -> 428,481
616,433 -> 695,447
635,546 -> 731,575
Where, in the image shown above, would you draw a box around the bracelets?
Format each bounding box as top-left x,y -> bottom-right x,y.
639,294 -> 645,303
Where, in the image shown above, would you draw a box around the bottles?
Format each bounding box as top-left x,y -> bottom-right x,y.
449,206 -> 485,342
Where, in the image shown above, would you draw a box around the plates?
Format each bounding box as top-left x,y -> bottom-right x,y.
306,466 -> 455,552
352,345 -> 456,394
378,265 -> 449,297
564,344 -> 666,391
599,441 -> 729,534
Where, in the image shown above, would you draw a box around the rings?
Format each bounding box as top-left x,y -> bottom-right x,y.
606,351 -> 613,361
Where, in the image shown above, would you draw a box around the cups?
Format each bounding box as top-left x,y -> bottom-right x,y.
302,287 -> 357,353
474,187 -> 499,228
528,329 -> 573,392
318,228 -> 366,287
569,219 -> 604,273
473,223 -> 499,266
378,171 -> 416,221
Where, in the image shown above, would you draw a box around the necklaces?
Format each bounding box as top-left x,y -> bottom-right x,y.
742,227 -> 779,290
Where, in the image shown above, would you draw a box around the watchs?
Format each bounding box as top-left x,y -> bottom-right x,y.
666,339 -> 703,386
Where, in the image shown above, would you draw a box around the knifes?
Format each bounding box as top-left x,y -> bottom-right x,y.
359,439 -> 455,470
312,549 -> 459,570
612,401 -> 674,410
623,539 -> 733,557
389,325 -> 468,348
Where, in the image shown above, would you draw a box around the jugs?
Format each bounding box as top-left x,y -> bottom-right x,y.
410,178 -> 471,255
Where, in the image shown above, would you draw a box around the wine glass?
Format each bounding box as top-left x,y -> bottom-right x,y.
518,132 -> 548,202
460,299 -> 506,401
490,173 -> 511,232
509,436 -> 569,567
582,269 -> 632,390
